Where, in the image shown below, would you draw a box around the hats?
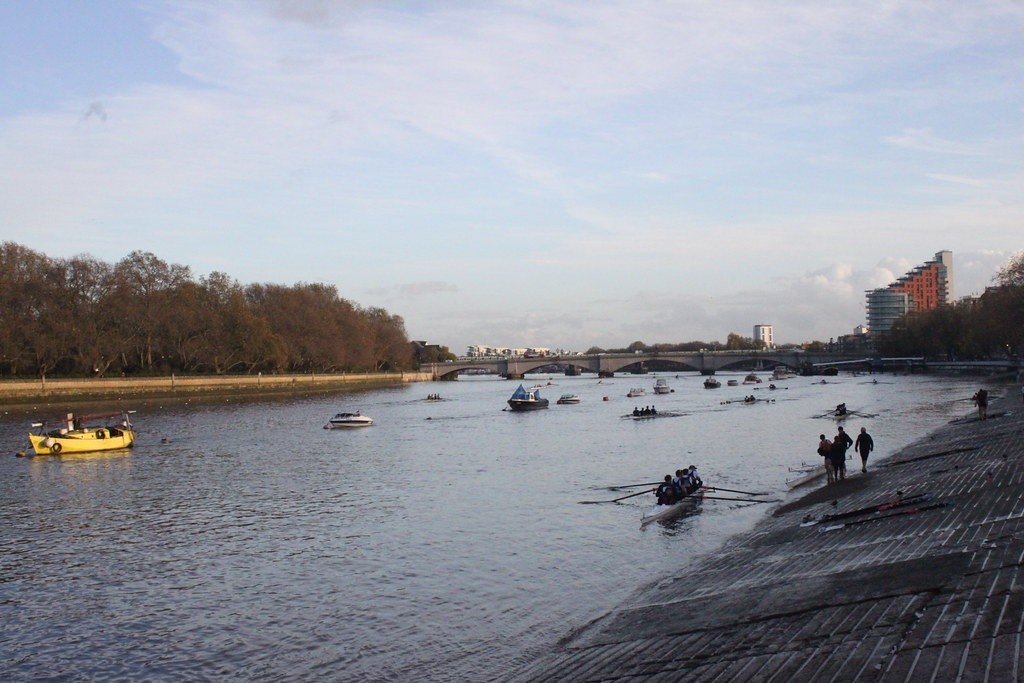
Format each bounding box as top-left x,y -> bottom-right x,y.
689,465 -> 697,469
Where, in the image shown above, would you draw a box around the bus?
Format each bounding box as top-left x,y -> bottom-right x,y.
524,352 -> 544,358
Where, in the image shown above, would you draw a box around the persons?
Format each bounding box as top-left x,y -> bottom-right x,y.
855,428 -> 873,473
633,405 -> 656,415
977,389 -> 987,421
121,420 -> 132,427
656,465 -> 702,505
428,393 -> 440,399
745,395 -> 755,402
769,384 -> 775,388
836,403 -> 846,416
817,426 -> 853,480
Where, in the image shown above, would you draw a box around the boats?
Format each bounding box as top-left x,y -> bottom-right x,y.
653,378 -> 670,394
627,387 -> 646,397
328,412 -> 373,428
831,412 -> 848,420
742,369 -> 762,385
771,365 -> 788,380
727,380 -> 738,386
741,399 -> 757,405
557,394 -> 580,405
703,381 -> 722,389
639,482 -> 704,524
506,383 -> 549,411
785,460 -> 828,488
28,409 -> 139,457
624,414 -> 656,420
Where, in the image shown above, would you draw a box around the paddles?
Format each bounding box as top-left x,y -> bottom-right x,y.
699,483 -> 769,497
612,487 -> 659,502
684,495 -> 767,503
818,409 -> 837,419
616,482 -> 667,489
848,412 -> 869,419
845,409 -> 871,416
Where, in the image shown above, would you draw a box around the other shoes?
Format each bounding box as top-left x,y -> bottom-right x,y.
862,468 -> 867,473
